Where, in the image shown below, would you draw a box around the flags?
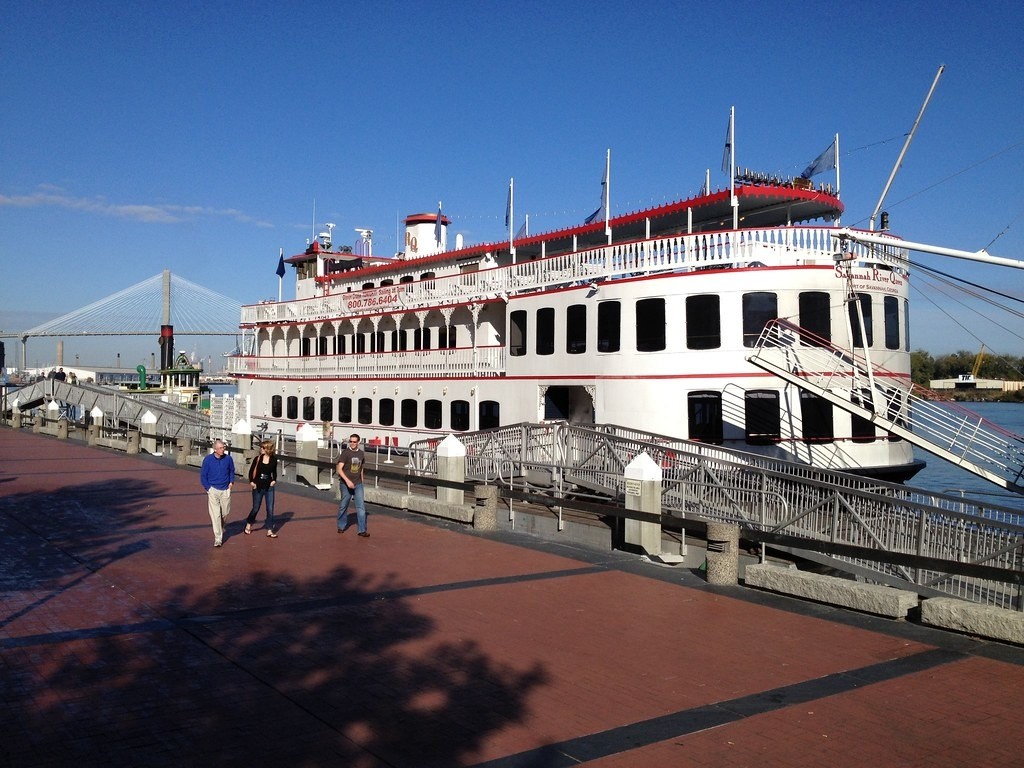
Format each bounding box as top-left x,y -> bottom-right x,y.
600,157 -> 607,209
585,207 -> 604,223
721,115 -> 733,175
275,255 -> 286,278
801,141 -> 836,178
513,222 -> 526,239
434,210 -> 441,247
505,185 -> 511,226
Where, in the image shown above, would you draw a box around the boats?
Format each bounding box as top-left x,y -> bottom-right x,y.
220,59 -> 1023,514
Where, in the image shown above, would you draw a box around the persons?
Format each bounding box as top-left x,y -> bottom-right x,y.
36,372 -> 45,382
47,368 -> 56,380
201,440 -> 235,546
244,442 -> 278,538
54,368 -> 66,381
64,372 -> 73,382
68,373 -> 79,386
337,433 -> 370,537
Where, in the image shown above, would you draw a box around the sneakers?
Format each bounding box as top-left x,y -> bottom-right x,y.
221,526 -> 227,533
213,543 -> 221,547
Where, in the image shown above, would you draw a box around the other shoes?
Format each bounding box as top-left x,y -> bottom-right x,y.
358,531 -> 370,537
243,524 -> 250,534
337,527 -> 344,533
265,530 -> 277,537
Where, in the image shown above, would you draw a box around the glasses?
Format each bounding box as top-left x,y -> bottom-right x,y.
348,439 -> 358,444
